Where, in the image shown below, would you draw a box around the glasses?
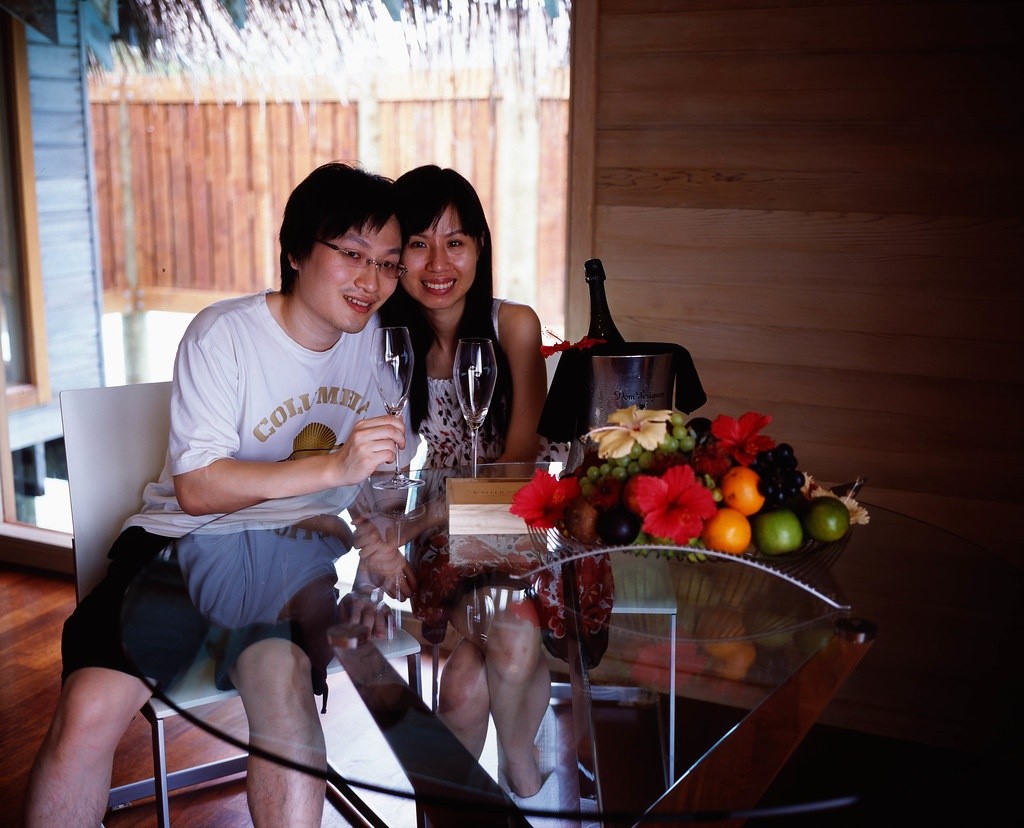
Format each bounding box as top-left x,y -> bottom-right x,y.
311,238 -> 407,280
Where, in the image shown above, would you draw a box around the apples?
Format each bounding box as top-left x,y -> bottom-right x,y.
803,495 -> 848,541
753,509 -> 804,555
592,476 -> 625,510
748,594 -> 796,647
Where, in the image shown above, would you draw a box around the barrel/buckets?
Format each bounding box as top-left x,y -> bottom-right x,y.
565,351 -> 680,474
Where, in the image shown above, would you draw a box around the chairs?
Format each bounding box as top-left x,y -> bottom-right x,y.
59,381 -> 422,828
419,347 -> 681,798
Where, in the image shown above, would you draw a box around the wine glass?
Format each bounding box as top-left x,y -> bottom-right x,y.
369,327 -> 427,490
371,496 -> 425,552
453,337 -> 498,479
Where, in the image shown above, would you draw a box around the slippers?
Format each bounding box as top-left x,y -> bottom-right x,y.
497,703 -> 559,828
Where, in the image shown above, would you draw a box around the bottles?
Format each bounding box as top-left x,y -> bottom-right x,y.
582,257 -> 627,356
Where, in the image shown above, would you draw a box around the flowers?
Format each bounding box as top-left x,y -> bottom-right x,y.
799,471 -> 873,528
709,410 -> 781,464
578,402 -> 671,460
507,468 -> 577,529
539,332 -> 608,360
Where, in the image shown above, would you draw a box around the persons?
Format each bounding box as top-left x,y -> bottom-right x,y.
348,166 -> 561,828
21,162 -> 422,828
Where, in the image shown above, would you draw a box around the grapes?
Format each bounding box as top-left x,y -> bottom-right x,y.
754,443 -> 807,512
576,412 -> 696,493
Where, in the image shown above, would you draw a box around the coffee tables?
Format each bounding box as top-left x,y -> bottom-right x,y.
120,461 -> 1023,828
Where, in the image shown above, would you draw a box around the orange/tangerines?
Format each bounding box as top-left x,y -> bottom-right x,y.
721,466 -> 770,517
694,571 -> 755,678
702,509 -> 752,554
796,616 -> 835,654
